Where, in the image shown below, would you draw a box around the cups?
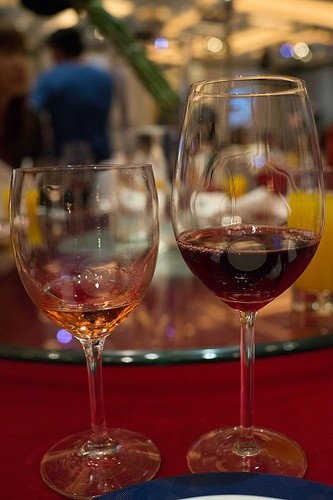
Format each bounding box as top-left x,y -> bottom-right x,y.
283,165 -> 333,316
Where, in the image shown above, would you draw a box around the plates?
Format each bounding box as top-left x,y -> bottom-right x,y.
85,473 -> 333,500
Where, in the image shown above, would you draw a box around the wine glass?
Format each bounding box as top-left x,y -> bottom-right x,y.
172,75 -> 325,478
9,165 -> 161,499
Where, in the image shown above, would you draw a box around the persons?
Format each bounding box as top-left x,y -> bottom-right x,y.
0,27 -> 35,117
28,28 -> 117,165
